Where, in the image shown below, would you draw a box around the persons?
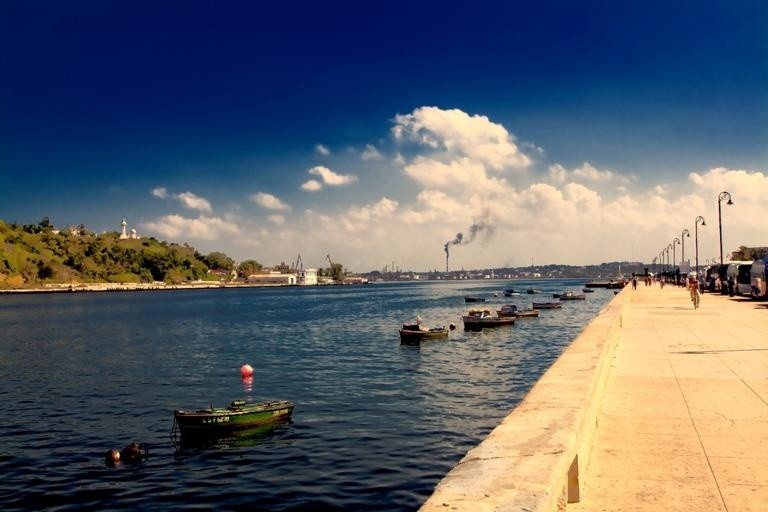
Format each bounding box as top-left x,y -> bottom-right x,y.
631,274 -> 763,311
119,441 -> 143,466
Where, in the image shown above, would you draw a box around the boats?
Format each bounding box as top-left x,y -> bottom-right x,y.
552,291 -> 585,300
398,316 -> 450,345
460,308 -> 516,331
462,296 -> 487,301
494,286 -> 541,296
172,400 -> 295,438
582,288 -> 593,293
172,424 -> 293,465
531,297 -> 561,309
492,307 -> 539,317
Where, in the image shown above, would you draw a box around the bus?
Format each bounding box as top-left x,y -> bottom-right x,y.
725,260 -> 751,294
652,264 -> 726,294
749,258 -> 768,299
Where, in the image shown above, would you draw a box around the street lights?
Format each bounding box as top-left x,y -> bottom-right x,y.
651,247 -> 666,273
681,228 -> 689,261
672,237 -> 680,268
717,190 -> 733,265
666,243 -> 672,266
695,215 -> 707,270
